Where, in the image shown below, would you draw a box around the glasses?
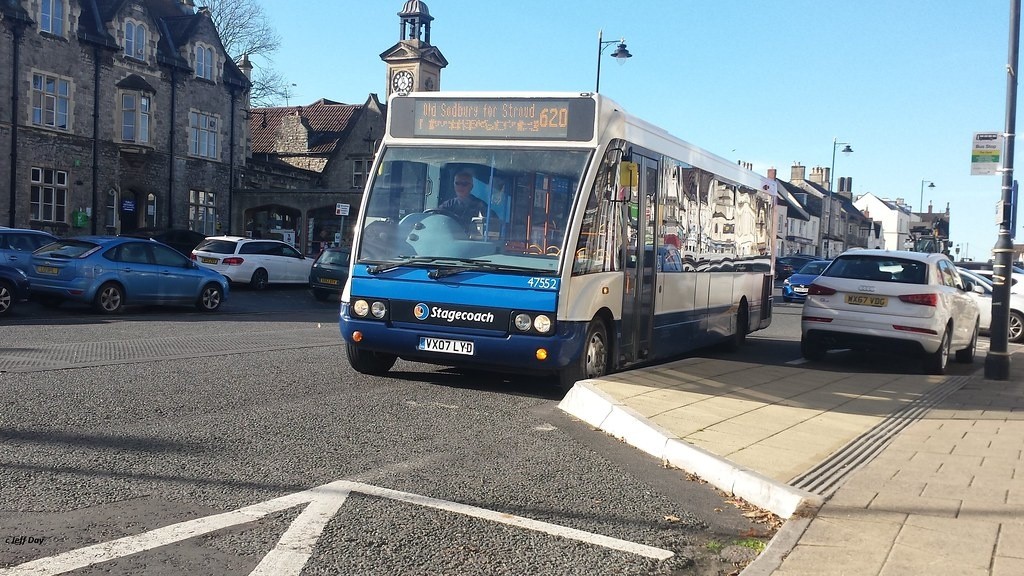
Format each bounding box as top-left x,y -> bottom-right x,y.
454,180 -> 472,185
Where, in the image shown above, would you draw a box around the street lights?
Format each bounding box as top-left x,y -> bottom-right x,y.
149,192 -> 157,228
825,136 -> 854,260
592,28 -> 634,92
919,180 -> 934,224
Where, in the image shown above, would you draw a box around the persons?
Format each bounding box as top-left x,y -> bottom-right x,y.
438,170 -> 499,237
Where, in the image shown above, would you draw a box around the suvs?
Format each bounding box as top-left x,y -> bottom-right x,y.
800,247 -> 982,373
189,236 -> 320,291
311,246 -> 350,298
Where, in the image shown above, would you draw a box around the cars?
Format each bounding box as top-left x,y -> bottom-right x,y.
0,265 -> 30,313
115,225 -> 210,257
773,255 -> 1024,343
0,227 -> 61,271
27,234 -> 229,315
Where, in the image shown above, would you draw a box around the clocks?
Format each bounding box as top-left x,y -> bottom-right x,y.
391,70 -> 414,92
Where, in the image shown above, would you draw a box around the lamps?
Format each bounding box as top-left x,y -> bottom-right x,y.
240,108 -> 268,128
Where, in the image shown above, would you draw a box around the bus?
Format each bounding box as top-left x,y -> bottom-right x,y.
335,90 -> 780,399
